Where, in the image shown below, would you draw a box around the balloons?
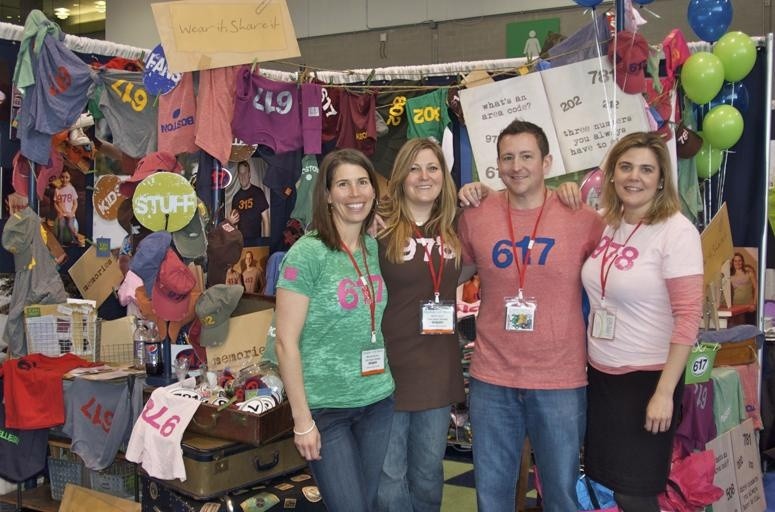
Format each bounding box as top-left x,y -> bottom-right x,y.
713,30 -> 759,84
686,0 -> 734,46
702,102 -> 745,150
680,50 -> 726,108
709,78 -> 750,120
690,131 -> 725,179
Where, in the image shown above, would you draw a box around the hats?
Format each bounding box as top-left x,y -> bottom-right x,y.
135,286 -> 168,342
168,264 -> 206,344
193,283 -> 246,349
150,247 -> 198,323
119,151 -> 186,197
12,148 -> 31,196
207,218 -> 244,286
36,148 -> 64,200
118,270 -> 144,319
606,31 -> 703,160
128,229 -> 172,300
171,196 -> 210,260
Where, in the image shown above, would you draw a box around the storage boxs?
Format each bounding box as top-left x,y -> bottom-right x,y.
45,436 -> 141,507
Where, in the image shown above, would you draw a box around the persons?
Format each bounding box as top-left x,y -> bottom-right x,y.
260,148 -> 397,512
224,263 -> 244,284
364,137 -> 499,512
455,116 -> 611,511
555,130 -> 706,512
729,252 -> 757,307
242,250 -> 264,292
228,159 -> 270,239
52,165 -> 81,242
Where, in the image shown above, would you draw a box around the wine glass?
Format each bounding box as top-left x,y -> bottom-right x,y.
174,357 -> 191,388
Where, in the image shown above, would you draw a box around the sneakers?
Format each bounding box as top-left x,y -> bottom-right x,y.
70,128 -> 91,146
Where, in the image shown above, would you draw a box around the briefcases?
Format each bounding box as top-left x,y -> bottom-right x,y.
137,429 -> 307,501
140,466 -> 325,512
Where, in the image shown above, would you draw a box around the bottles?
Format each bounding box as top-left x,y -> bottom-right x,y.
133,320 -> 147,368
144,322 -> 164,377
197,362 -> 234,402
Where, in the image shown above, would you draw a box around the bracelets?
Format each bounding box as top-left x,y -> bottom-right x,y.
292,419 -> 317,437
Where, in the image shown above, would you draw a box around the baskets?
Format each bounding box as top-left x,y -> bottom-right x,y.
49,439 -> 125,465
48,457 -> 136,502
